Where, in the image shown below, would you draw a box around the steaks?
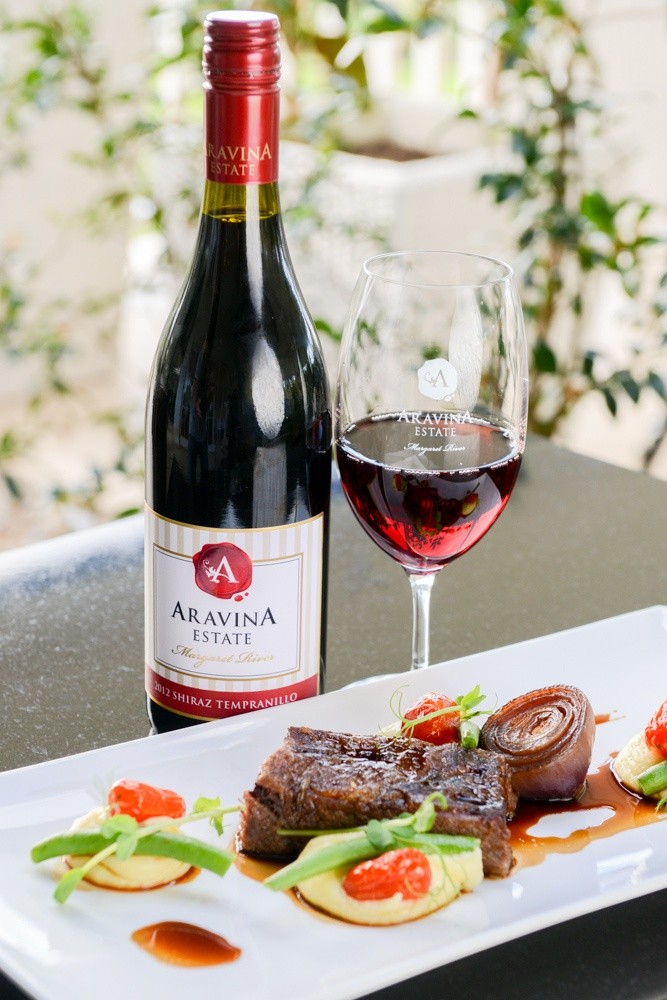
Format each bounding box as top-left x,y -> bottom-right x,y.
237,726 -> 523,884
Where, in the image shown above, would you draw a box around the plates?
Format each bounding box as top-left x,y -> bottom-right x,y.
1,606 -> 666,999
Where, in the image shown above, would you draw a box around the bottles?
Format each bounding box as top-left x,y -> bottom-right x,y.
141,7 -> 339,733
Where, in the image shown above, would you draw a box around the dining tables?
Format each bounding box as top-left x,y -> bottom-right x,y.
1,437 -> 667,998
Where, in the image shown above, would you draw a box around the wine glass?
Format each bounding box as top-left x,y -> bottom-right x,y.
333,243 -> 532,691
